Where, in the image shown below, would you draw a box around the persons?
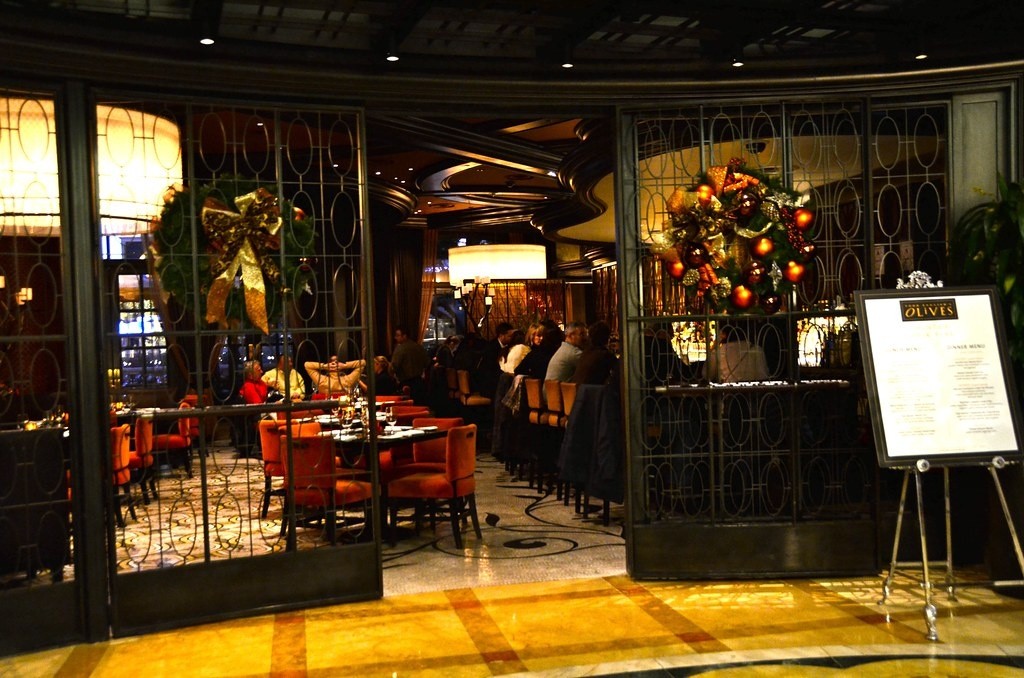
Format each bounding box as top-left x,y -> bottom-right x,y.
239,353 -> 400,404
392,319 -> 736,385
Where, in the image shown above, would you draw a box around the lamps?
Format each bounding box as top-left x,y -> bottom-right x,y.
447,207 -> 547,280
449,280 -> 496,307
0,96 -> 184,237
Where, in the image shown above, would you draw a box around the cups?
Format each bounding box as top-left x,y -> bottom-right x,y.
42,410 -> 54,428
54,405 -> 65,428
18,414 -> 28,430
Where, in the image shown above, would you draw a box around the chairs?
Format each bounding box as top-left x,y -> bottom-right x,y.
504,376 -> 622,528
64,394 -> 210,528
259,394 -> 482,548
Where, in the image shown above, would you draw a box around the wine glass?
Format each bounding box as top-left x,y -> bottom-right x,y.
342,415 -> 353,441
386,406 -> 397,435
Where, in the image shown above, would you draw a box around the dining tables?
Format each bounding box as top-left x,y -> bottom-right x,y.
312,408 -> 393,425
315,423 -> 448,548
